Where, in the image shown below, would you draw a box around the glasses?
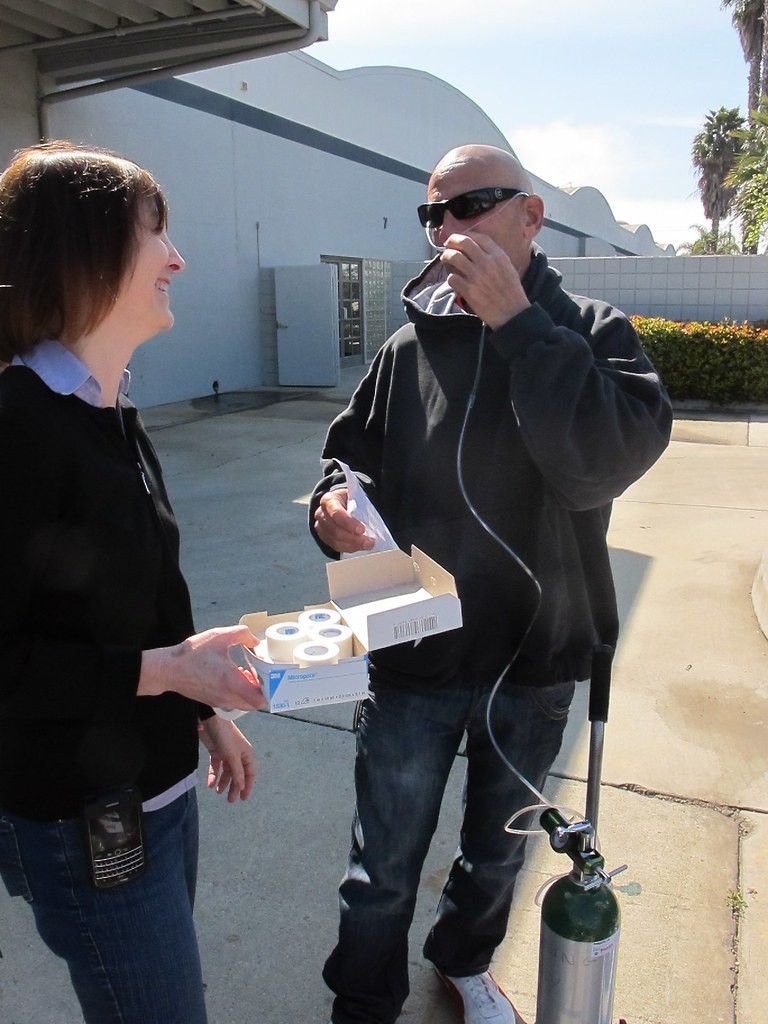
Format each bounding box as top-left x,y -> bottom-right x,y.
417,187 -> 528,228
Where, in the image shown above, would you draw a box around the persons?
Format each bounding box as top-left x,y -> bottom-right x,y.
2,144 -> 269,1024
306,140 -> 679,1023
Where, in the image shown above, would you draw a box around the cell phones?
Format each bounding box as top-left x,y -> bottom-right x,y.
84,789 -> 146,888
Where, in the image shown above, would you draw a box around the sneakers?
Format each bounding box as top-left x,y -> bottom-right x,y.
432,962 -> 516,1024
